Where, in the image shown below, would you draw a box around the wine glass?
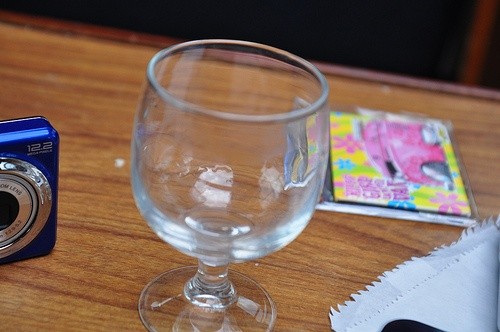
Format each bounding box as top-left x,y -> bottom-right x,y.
129,38 -> 331,331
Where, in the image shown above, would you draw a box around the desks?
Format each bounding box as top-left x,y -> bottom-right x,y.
0,11 -> 500,332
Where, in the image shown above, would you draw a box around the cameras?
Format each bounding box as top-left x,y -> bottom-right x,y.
0,116 -> 59,267
